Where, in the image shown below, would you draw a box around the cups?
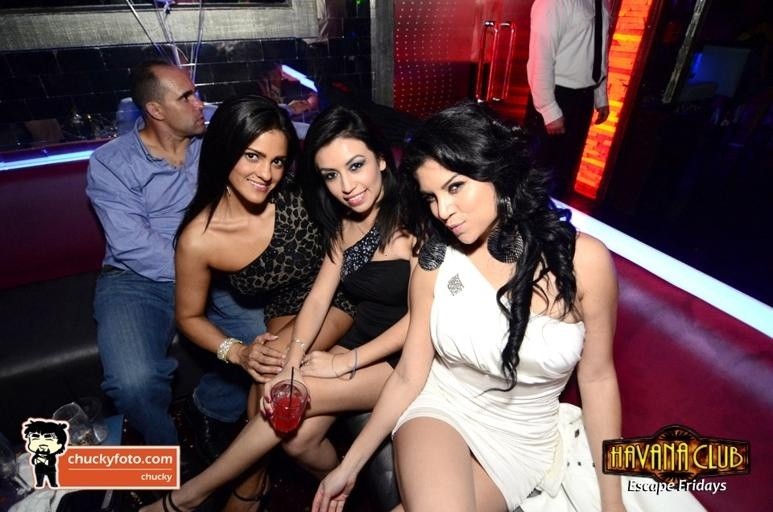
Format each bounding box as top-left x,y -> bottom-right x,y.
270,378 -> 308,432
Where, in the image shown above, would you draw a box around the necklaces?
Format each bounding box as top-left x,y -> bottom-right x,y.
357,224 -> 365,235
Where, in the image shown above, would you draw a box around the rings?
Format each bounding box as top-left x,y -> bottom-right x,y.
256,336 -> 264,344
304,362 -> 309,366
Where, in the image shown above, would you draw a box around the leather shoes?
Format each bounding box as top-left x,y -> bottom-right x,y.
185,392 -> 216,444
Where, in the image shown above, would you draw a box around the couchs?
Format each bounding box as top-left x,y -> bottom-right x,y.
1,122 -> 773,511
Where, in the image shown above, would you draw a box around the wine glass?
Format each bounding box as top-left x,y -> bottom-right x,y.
0,432 -> 35,496
52,403 -> 102,446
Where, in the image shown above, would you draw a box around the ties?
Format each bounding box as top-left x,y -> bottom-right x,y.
591,0 -> 603,83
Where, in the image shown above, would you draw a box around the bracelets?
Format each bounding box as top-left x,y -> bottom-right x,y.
216,337 -> 242,364
332,353 -> 349,381
350,347 -> 357,379
286,338 -> 305,351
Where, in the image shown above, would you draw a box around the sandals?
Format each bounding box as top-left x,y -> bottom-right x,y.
222,466 -> 274,512
138,490 -> 183,512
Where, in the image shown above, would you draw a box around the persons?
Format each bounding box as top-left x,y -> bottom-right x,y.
311,103 -> 627,512
85,59 -> 268,445
115,44 -> 320,136
138,103 -> 424,512
172,94 -> 359,512
524,0 -> 610,199
